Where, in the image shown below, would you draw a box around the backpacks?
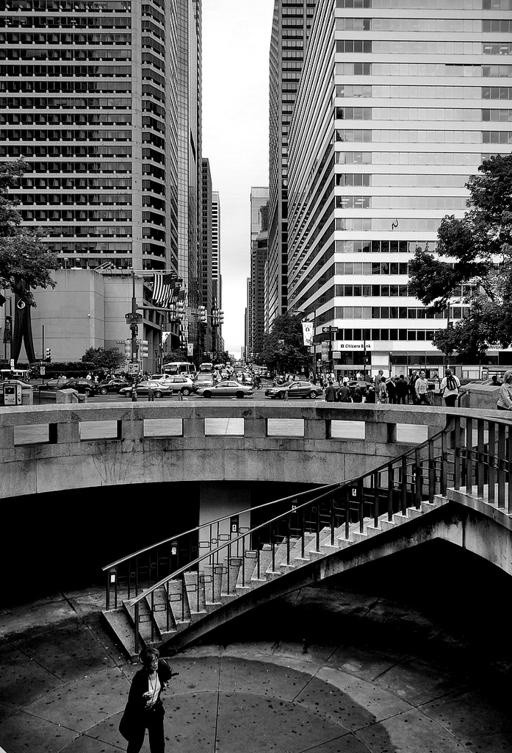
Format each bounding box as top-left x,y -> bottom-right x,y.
447,375 -> 458,390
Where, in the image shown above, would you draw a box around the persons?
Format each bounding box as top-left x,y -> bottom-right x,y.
491,376 -> 501,386
497,370 -> 512,411
119,648 -> 173,752
440,368 -> 460,406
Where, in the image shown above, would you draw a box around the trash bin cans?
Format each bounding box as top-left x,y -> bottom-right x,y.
3,383 -> 22,406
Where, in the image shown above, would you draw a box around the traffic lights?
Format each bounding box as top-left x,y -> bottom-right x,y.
139,339 -> 149,358
175,301 -> 184,319
216,309 -> 225,326
196,305 -> 206,322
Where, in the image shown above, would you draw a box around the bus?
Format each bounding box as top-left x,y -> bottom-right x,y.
161,360 -> 196,381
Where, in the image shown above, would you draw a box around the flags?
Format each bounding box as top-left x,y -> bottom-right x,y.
152,272 -> 183,307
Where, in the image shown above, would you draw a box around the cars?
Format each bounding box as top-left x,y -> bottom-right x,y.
326,379 -> 375,396
265,381 -> 323,399
198,360 -> 269,387
42,372 -> 195,399
196,381 -> 255,398
0,379 -> 34,398
